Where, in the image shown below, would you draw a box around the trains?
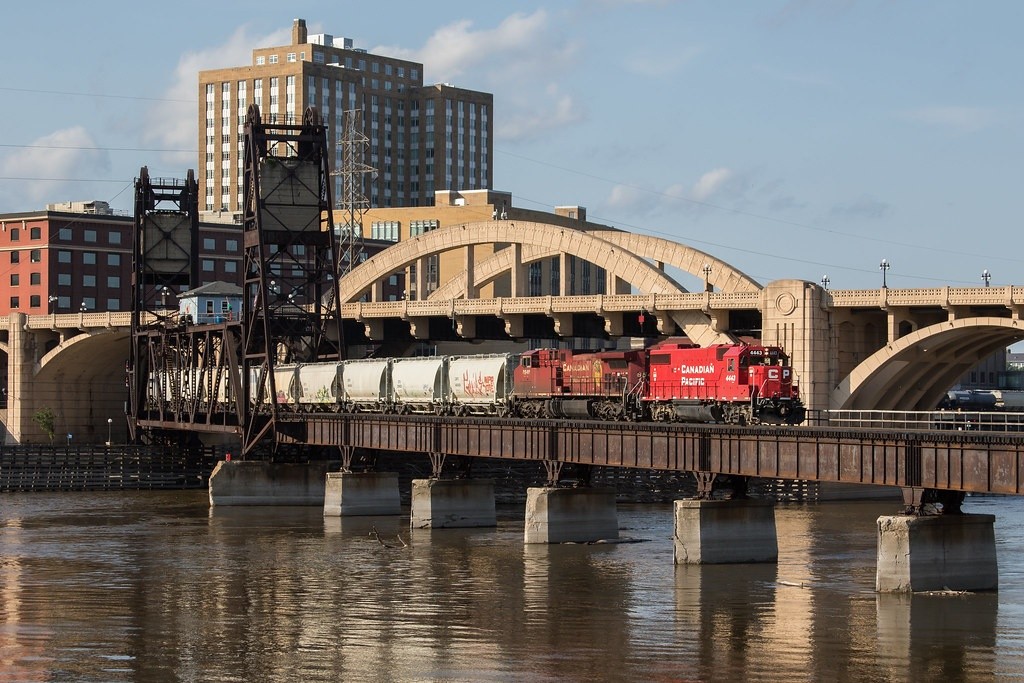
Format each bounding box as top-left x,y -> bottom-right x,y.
141,336 -> 807,429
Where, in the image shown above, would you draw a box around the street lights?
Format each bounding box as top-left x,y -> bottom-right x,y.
879,258 -> 890,288
701,264 -> 711,292
981,269 -> 991,289
268,280 -> 278,309
821,275 -> 830,291
48,294 -> 58,315
107,418 -> 112,442
